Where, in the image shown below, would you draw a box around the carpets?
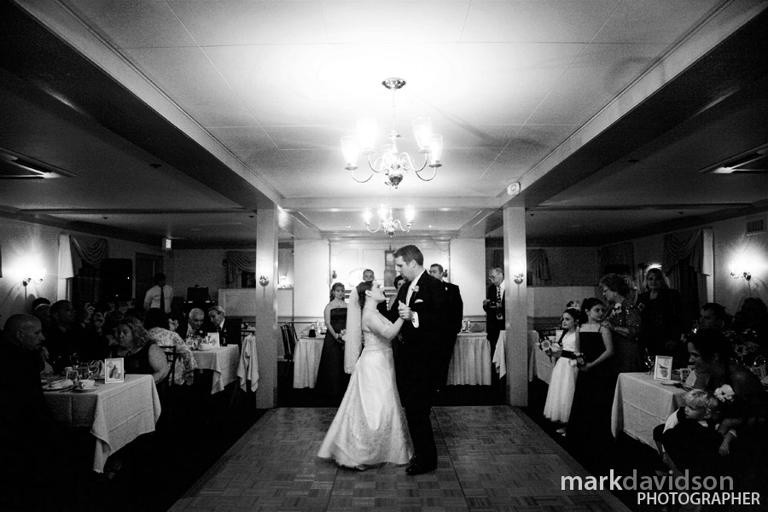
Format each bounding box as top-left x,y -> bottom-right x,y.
166,405 -> 627,511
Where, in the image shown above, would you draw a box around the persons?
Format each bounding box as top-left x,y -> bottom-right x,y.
360,268 -> 388,313
143,272 -> 175,315
355,280 -> 408,472
379,243 -> 452,478
2,283 -> 244,484
315,282 -> 349,403
482,267 -> 506,395
544,262 -> 768,511
385,274 -> 406,309
426,264 -> 463,400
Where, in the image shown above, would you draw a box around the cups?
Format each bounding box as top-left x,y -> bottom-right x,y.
185,334 -> 215,352
43,374 -> 101,392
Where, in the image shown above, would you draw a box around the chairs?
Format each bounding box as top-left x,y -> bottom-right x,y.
280,322 -> 298,377
158,344 -> 178,428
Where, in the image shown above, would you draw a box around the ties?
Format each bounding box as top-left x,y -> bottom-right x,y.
497,287 -> 501,317
160,287 -> 165,312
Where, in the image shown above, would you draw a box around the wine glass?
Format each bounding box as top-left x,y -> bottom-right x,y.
644,355 -> 655,378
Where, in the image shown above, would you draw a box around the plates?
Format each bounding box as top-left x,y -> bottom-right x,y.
660,380 -> 682,386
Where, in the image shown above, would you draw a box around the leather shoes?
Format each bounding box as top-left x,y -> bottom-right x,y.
406,455 -> 437,475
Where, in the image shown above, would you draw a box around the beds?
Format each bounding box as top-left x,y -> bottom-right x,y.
183,342 -> 239,394
